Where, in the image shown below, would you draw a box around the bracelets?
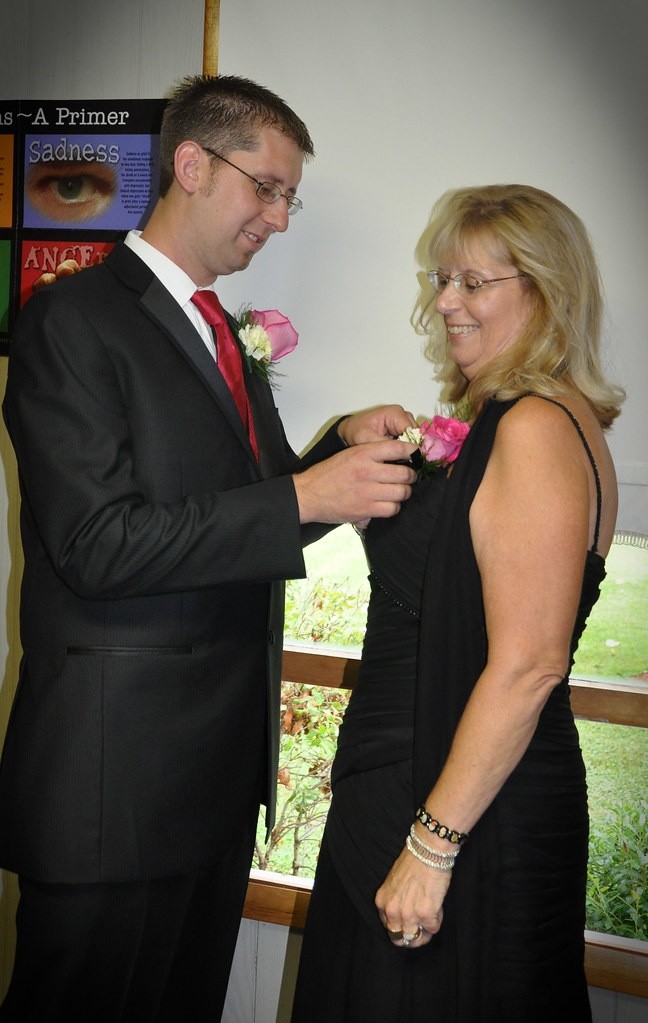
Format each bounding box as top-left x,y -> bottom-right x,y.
406,824 -> 459,869
416,806 -> 470,845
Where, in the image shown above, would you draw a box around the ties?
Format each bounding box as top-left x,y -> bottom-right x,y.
191,290 -> 259,461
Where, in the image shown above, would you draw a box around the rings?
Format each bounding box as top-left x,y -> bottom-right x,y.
403,927 -> 421,945
386,922 -> 403,940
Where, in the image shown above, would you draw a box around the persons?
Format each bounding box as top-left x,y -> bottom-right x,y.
1,76 -> 420,1023
290,183 -> 627,1023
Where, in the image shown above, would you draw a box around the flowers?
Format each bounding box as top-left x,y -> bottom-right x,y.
395,411 -> 470,484
229,300 -> 300,390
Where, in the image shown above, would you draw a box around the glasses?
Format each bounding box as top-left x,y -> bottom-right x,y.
199,147 -> 304,215
425,269 -> 529,299
